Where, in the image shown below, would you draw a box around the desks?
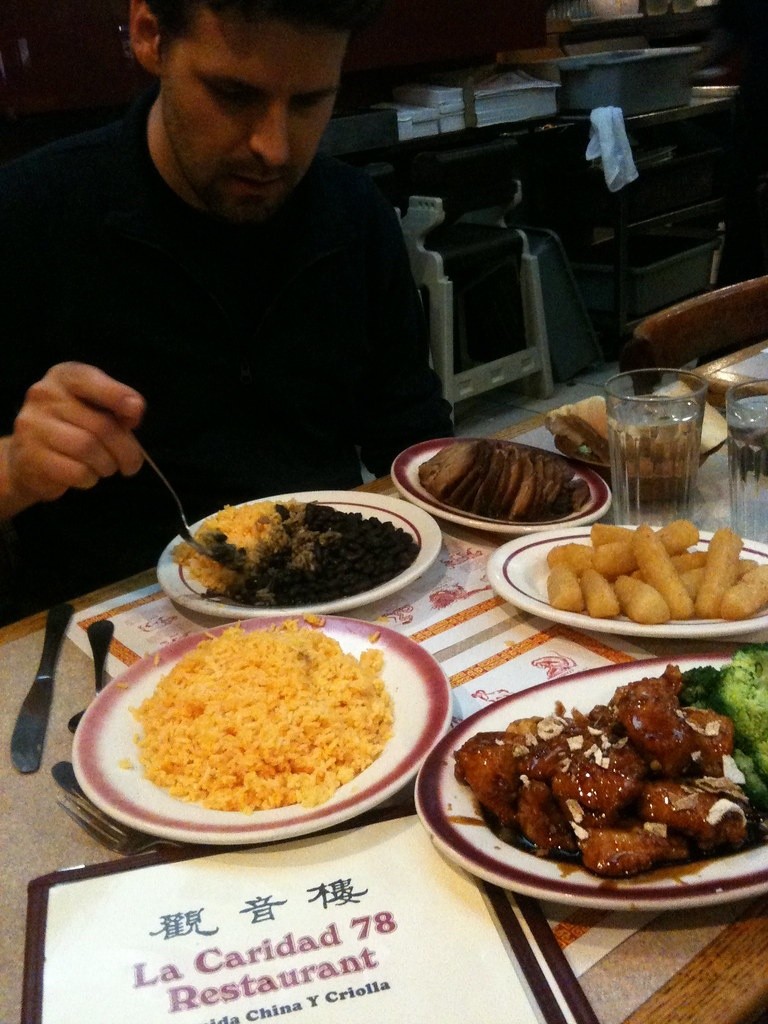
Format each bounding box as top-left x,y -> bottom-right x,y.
1,335 -> 768,1023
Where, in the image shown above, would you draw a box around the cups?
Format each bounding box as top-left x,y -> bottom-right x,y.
724,378 -> 768,548
603,369 -> 707,535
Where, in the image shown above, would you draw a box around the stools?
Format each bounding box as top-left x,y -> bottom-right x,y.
392,179 -> 553,424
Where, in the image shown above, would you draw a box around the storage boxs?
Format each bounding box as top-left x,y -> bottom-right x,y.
555,45 -> 701,114
317,108 -> 401,156
572,235 -> 719,315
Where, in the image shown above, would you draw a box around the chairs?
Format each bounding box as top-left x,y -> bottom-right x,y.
624,273 -> 768,392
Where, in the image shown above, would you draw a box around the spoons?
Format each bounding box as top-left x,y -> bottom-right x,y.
68,620 -> 115,733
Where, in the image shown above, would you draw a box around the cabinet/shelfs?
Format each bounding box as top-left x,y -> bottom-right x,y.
562,99 -> 736,341
0,1 -> 545,119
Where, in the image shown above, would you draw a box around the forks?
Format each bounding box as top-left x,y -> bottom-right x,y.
115,420 -> 227,558
56,787 -> 201,857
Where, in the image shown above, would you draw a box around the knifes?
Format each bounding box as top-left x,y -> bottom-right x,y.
10,602 -> 76,773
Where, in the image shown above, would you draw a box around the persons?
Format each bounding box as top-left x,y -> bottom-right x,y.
0,0 -> 453,627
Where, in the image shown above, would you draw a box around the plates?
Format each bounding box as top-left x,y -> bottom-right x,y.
156,491 -> 443,617
486,525 -> 768,637
72,615 -> 453,846
390,438 -> 613,534
554,434 -> 728,483
415,651 -> 768,911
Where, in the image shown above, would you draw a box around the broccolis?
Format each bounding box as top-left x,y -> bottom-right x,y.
677,645 -> 768,816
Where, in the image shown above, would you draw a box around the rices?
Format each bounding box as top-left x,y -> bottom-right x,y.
170,498 -> 309,595
115,616 -> 394,812
